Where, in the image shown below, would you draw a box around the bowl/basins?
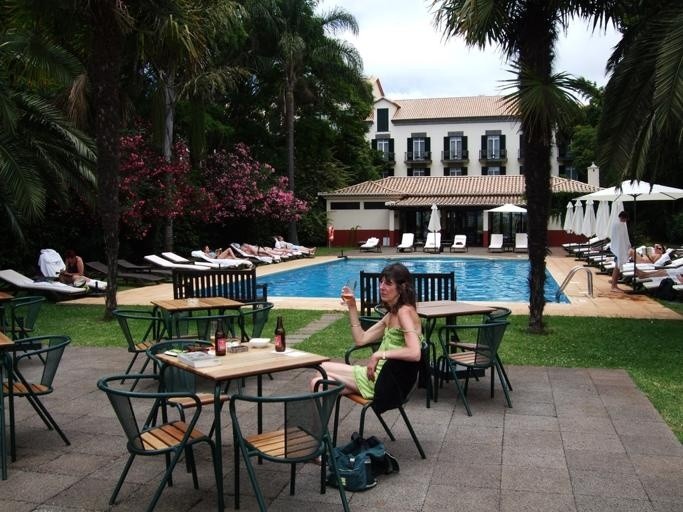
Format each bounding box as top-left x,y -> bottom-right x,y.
249,337 -> 270,347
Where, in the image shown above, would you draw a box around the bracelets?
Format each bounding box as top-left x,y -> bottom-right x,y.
382,350 -> 386,360
350,322 -> 361,327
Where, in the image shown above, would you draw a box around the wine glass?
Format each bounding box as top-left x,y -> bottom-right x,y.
342,279 -> 358,306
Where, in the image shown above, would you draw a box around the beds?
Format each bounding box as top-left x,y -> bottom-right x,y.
41,250 -> 115,296
273,240 -> 316,262
162,251 -> 234,274
143,254 -> 212,281
574,242 -> 612,263
397,232 -> 415,253
1,268 -> 87,304
633,270 -> 683,296
450,235 -> 468,252
360,237 -> 382,252
249,245 -> 281,265
229,242 -> 271,267
600,246 -> 665,279
488,233 -> 504,252
514,232 -> 531,254
562,236 -> 604,249
86,261 -> 164,291
423,232 -> 442,253
594,247 -> 648,270
191,250 -> 255,271
587,248 -> 627,266
275,248 -> 293,261
118,259 -> 178,285
620,257 -> 683,285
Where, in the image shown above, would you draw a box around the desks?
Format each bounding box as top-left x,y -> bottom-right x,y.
390,299 -> 497,403
1,334 -> 20,481
0,288 -> 14,332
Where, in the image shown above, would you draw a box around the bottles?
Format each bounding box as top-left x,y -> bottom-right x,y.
275,315 -> 286,351
215,318 -> 227,356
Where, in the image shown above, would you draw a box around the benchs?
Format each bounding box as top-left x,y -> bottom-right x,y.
358,270 -> 459,331
169,264 -> 269,326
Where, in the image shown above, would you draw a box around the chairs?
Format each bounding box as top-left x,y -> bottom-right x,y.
112,309 -> 180,391
344,339 -> 430,460
374,300 -> 394,322
95,374 -> 226,511
145,336 -> 243,473
3,334 -> 75,450
359,314 -> 435,395
230,300 -> 275,389
177,313 -> 242,389
434,320 -> 512,421
227,380 -> 351,512
450,306 -> 512,390
2,293 -> 49,368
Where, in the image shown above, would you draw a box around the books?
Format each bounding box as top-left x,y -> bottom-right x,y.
176,351 -> 221,369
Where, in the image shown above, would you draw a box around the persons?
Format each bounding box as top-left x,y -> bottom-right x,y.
58,250 -> 85,286
635,265 -> 683,283
609,210 -> 632,293
277,234 -> 311,253
264,246 -> 295,256
627,243 -> 666,264
306,262 -> 422,465
237,242 -> 275,259
202,244 -> 241,261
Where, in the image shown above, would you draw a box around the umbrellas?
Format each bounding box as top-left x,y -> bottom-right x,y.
596,201 -> 610,273
572,179 -> 683,294
484,203 -> 527,252
427,203 -> 441,254
580,201 -> 596,265
570,199 -> 584,259
563,201 -> 574,256
609,201 -> 625,225
